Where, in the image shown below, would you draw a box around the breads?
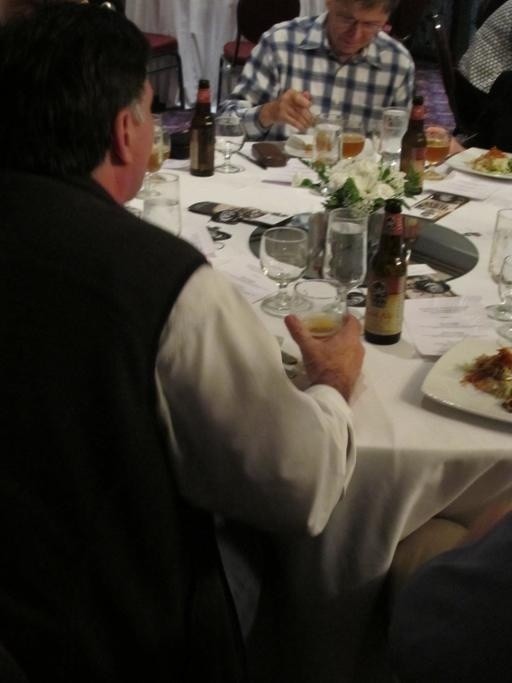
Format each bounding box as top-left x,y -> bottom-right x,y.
474,146 -> 512,174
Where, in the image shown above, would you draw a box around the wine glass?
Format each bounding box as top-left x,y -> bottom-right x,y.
211,116 -> 246,176
136,113 -> 171,196
260,225 -> 314,316
321,207 -> 366,314
485,208 -> 511,339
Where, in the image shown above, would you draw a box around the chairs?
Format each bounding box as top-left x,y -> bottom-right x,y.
217,0 -> 299,115
89,0 -> 184,111
430,8 -> 486,138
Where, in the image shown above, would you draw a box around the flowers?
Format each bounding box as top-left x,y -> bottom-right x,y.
292,147 -> 426,218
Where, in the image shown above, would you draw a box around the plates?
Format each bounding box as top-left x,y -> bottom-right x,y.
445,146 -> 511,180
421,333 -> 512,425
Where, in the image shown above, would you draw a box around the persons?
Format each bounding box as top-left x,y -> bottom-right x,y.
216,1 -> 511,153
3,1 -> 362,679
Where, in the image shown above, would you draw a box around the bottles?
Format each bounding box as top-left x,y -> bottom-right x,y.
362,198 -> 408,344
186,79 -> 220,176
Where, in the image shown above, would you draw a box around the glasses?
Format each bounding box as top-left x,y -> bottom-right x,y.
334,10 -> 385,36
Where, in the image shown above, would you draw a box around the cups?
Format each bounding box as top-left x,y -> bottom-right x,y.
311,94 -> 453,196
291,278 -> 348,339
142,171 -> 182,238
